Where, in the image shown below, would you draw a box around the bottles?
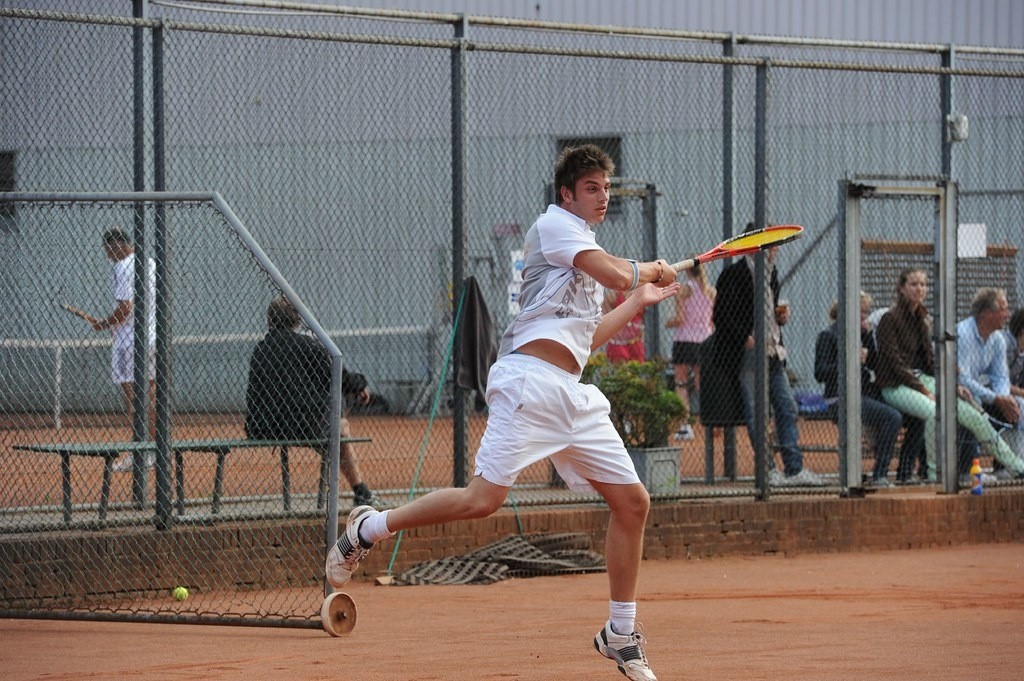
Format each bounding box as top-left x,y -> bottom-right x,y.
970,459 -> 982,496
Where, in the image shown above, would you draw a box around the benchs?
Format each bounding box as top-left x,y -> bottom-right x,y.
10,437 -> 374,521
768,393 -> 1009,483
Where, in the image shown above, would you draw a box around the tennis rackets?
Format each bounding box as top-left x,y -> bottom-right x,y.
60,302 -> 97,322
667,225 -> 804,272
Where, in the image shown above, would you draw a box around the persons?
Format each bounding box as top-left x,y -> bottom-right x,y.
934,286 -> 1023,487
93,228 -> 163,473
243,299 -> 391,510
663,254 -> 721,443
992,308 -> 1023,480
815,288 -> 922,488
324,146 -> 681,681
876,267 -> 1024,490
711,223 -> 832,488
604,284 -> 646,436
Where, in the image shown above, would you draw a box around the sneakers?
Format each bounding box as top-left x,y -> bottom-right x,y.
592,619 -> 658,681
325,505 -> 380,589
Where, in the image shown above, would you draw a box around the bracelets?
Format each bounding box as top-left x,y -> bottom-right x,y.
650,259 -> 665,284
624,255 -> 640,293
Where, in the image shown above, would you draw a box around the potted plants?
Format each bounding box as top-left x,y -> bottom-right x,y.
579,354 -> 689,498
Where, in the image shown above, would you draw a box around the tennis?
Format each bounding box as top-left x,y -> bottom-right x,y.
172,587 -> 189,602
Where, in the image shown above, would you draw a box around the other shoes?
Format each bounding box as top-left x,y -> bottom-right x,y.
352,494 -> 382,509
111,451 -> 165,473
673,424 -> 696,440
863,467 -> 1024,490
621,419 -> 634,437
768,467 -> 832,487
